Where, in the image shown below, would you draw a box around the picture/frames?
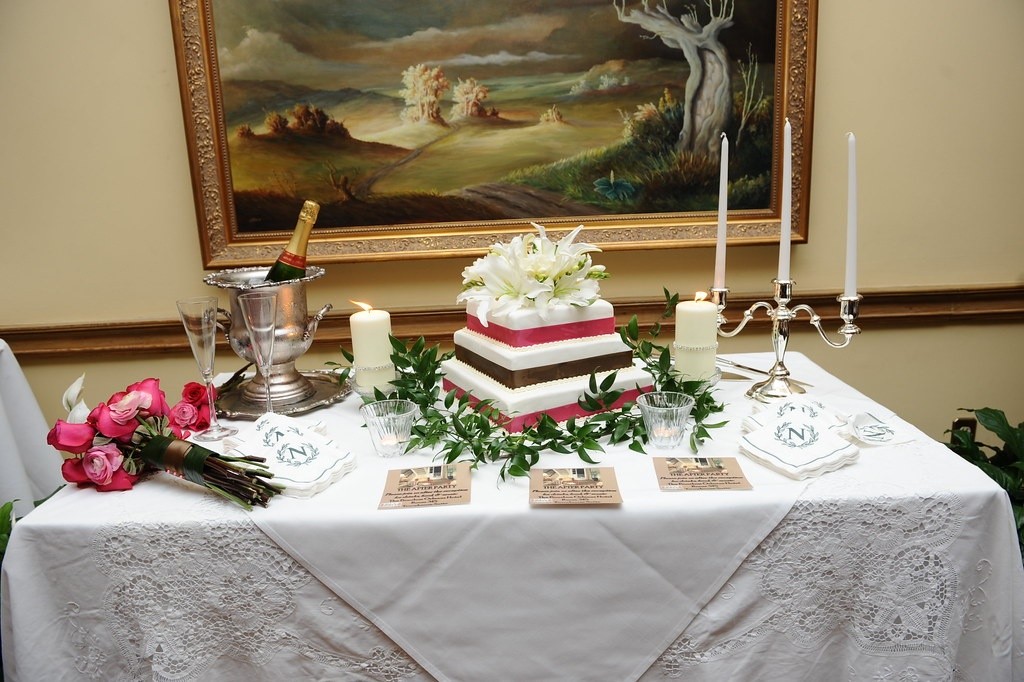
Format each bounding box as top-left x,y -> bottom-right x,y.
169,0 -> 818,271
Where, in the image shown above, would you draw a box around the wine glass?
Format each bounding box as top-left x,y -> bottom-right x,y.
176,296 -> 238,442
237,291 -> 278,412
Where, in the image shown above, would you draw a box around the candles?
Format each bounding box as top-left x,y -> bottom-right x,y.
674,291 -> 717,377
348,298 -> 393,386
842,132 -> 857,298
712,132 -> 729,289
777,118 -> 792,283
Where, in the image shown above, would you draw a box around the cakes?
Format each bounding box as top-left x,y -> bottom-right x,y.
441,221 -> 655,433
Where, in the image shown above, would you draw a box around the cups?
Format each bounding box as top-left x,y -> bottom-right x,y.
350,370 -> 401,403
359,399 -> 418,459
636,392 -> 695,448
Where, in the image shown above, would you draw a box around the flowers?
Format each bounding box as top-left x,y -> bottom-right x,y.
46,372 -> 285,511
455,221 -> 611,329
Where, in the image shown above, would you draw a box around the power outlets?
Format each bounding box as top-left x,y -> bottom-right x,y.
950,417 -> 977,445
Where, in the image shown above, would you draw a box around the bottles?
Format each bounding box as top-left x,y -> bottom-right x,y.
264,199 -> 320,284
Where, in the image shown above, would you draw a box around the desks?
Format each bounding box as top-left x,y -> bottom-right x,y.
0,350 -> 1024,682
